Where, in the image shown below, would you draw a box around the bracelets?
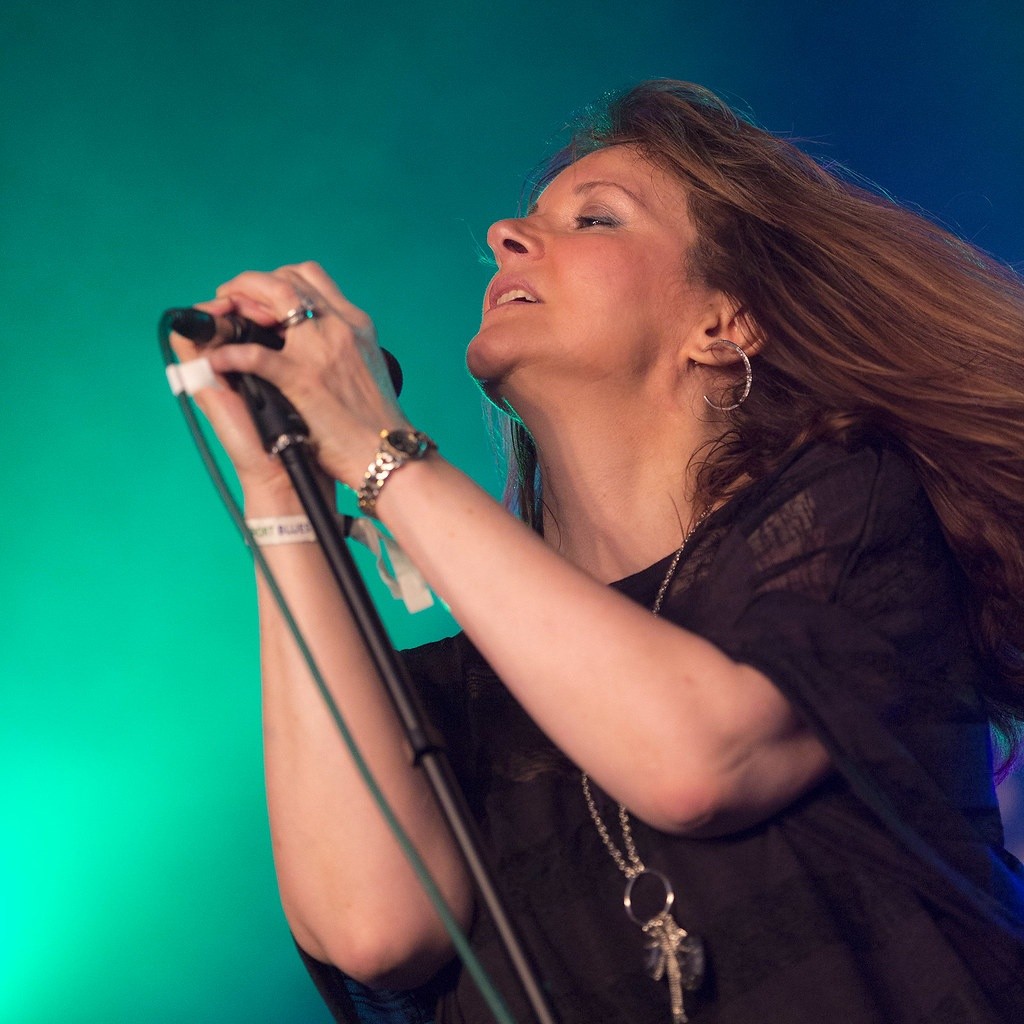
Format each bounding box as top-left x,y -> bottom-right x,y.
245,514 -> 434,615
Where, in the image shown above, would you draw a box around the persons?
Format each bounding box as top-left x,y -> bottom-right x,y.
169,79 -> 1024,1024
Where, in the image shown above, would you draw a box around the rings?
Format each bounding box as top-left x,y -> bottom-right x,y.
280,304 -> 319,327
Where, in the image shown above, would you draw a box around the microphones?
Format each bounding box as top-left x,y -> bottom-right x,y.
170,308 -> 403,399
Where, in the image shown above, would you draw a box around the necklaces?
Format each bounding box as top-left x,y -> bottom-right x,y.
581,503 -> 713,1024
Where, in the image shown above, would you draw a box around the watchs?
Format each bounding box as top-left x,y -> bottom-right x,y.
355,426 -> 438,519
